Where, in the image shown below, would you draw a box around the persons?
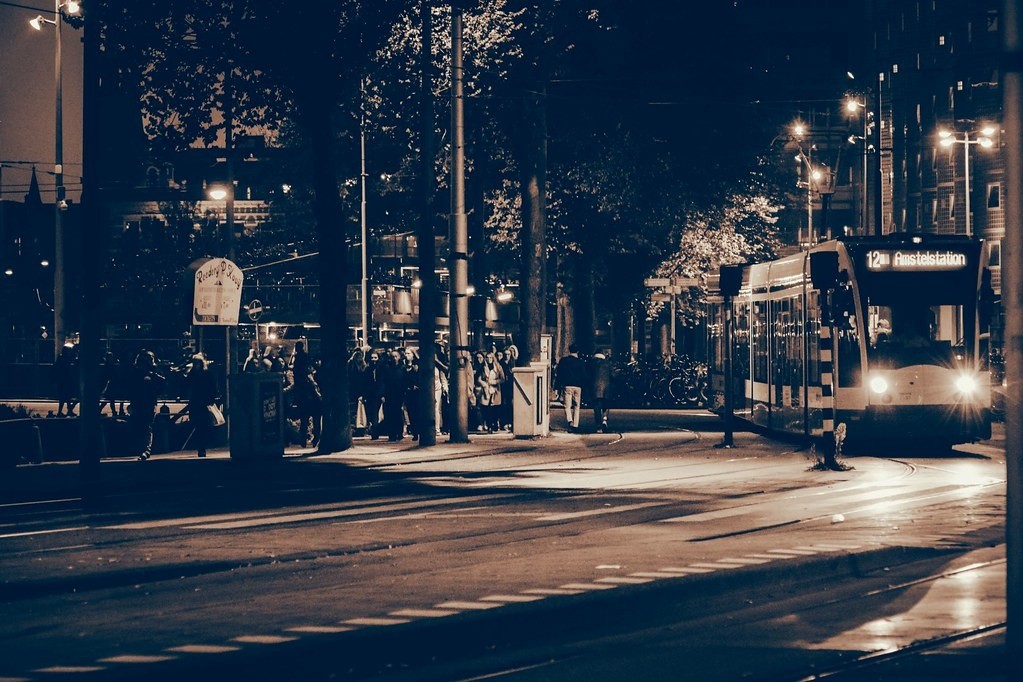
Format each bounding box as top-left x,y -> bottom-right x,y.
57,333 -> 520,458
587,345 -> 614,436
555,344 -> 591,433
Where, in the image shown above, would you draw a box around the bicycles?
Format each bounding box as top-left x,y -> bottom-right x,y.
602,345 -> 708,407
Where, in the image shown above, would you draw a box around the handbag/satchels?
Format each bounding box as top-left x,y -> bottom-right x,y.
378,403 -> 384,423
356,400 -> 367,428
207,403 -> 225,427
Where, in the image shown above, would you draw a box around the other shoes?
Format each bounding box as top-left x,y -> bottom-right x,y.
568,421 -> 574,432
500,426 -> 506,430
67,412 -> 77,416
112,412 -> 117,417
58,412 -> 66,417
197,449 -> 206,457
602,420 -> 608,432
101,413 -> 107,417
119,412 -> 126,415
413,436 -> 418,441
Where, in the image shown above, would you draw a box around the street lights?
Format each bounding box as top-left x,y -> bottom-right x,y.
937,123 -> 996,235
846,93 -> 868,235
205,66 -> 236,440
28,0 -> 90,364
786,121 -> 813,249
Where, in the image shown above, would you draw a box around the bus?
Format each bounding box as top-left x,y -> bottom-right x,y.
707,187 -> 993,446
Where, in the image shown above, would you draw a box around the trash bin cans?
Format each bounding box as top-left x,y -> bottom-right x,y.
229,370 -> 286,459
